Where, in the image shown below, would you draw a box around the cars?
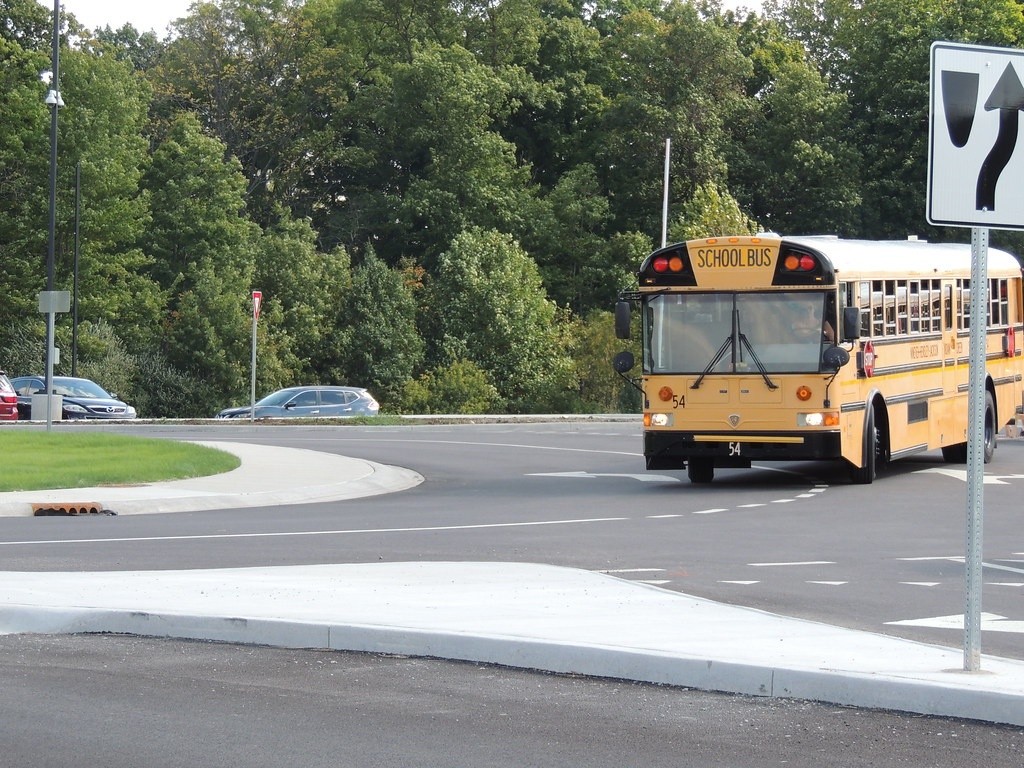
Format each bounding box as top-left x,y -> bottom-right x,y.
10,375 -> 138,420
0,370 -> 18,420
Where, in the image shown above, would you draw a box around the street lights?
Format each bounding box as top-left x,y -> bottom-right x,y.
41,0 -> 66,395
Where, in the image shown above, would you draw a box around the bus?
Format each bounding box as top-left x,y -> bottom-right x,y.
613,233 -> 1024,484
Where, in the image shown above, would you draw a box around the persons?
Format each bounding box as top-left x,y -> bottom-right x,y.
789,297 -> 834,344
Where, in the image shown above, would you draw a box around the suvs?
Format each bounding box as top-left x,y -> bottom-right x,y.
215,385 -> 379,420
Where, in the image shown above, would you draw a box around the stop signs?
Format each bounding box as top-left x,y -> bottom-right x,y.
863,339 -> 876,379
1006,327 -> 1016,357
